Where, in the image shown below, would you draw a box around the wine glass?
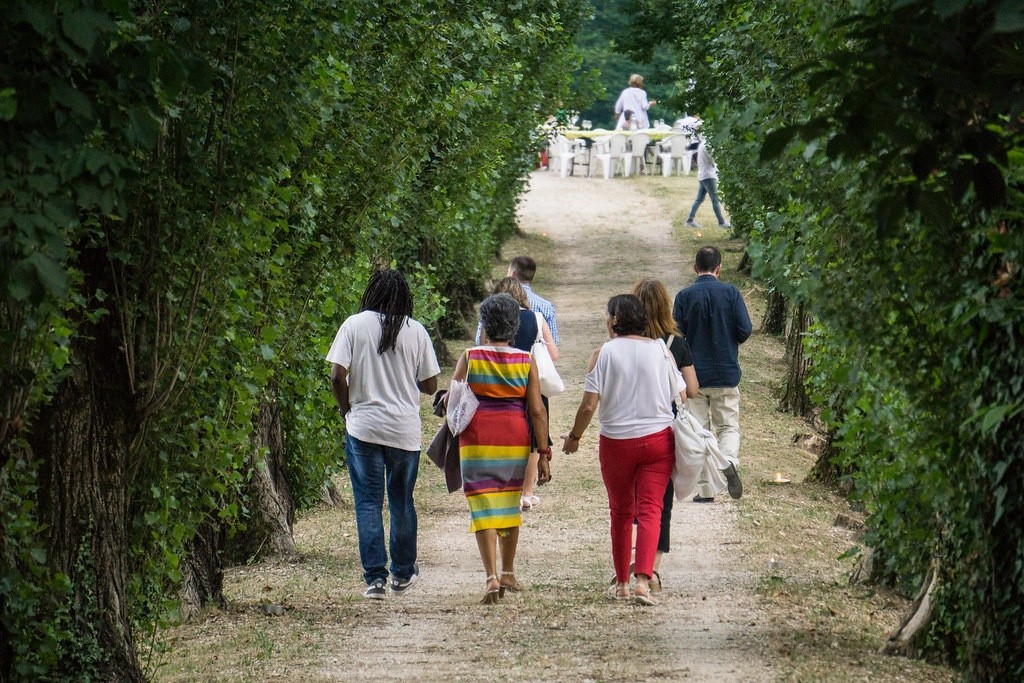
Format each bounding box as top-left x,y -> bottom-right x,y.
582,120 -> 592,131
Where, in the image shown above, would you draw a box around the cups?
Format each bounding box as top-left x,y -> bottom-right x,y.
654,119 -> 664,128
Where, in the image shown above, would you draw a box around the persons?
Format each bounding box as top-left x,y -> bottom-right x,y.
475,255 -> 558,348
673,247 -> 753,501
325,270 -> 441,601
685,140 -> 732,227
480,277 -> 559,511
561,294 -> 688,606
444,292 -> 551,603
614,73 -> 658,129
629,277 -> 698,591
621,109 -> 646,171
675,110 -> 704,164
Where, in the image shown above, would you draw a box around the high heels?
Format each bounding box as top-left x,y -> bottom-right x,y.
500,569 -> 522,599
479,575 -> 499,603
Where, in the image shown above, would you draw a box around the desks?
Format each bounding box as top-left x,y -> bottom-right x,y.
564,131 -> 673,176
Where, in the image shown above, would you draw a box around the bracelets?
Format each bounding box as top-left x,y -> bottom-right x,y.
537,448 -> 551,454
338,409 -> 348,415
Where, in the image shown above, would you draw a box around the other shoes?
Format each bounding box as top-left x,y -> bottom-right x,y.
686,219 -> 703,227
718,221 -> 733,227
648,570 -> 663,596
610,546 -> 636,584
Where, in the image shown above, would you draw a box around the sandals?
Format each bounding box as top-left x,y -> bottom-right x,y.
521,494 -> 540,510
634,584 -> 659,605
607,581 -> 631,600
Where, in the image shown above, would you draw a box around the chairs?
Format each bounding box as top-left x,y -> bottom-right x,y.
547,133 -> 698,179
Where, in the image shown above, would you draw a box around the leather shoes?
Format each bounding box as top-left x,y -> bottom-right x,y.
722,460 -> 743,499
693,493 -> 714,503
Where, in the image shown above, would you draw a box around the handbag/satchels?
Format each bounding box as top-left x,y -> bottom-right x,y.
530,312 -> 565,399
446,380 -> 480,437
671,409 -> 732,500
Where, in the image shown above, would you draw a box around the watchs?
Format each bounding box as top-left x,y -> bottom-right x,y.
569,432 -> 580,440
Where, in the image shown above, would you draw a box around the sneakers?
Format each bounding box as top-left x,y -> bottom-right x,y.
390,562 -> 420,594
363,578 -> 387,599
